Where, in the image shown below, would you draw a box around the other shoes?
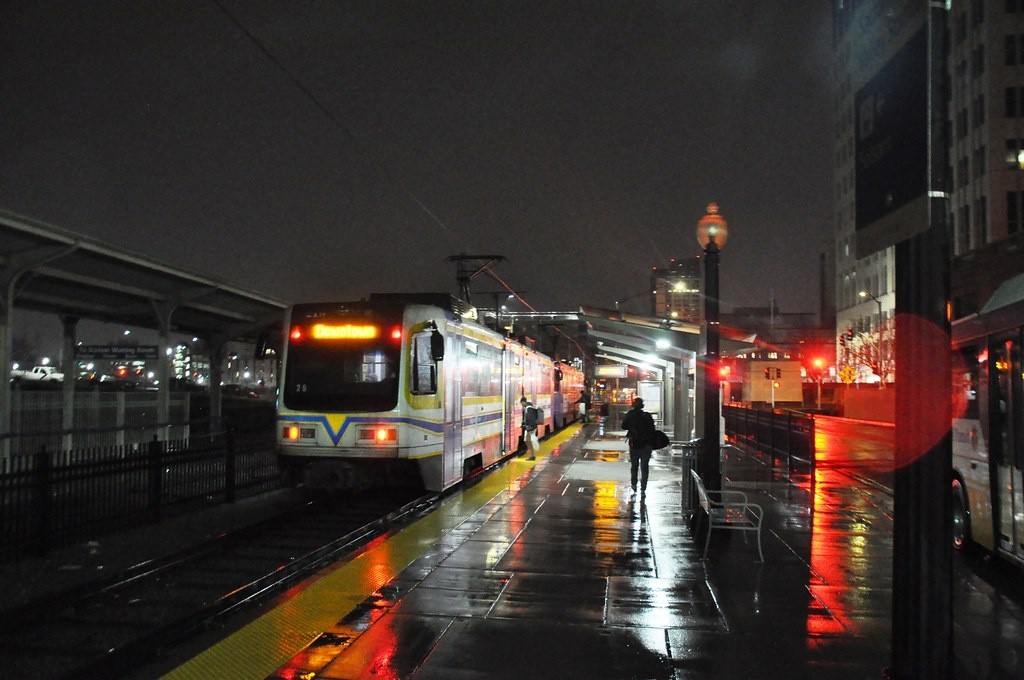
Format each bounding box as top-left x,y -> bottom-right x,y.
631,485 -> 637,492
528,457 -> 536,460
641,492 -> 646,498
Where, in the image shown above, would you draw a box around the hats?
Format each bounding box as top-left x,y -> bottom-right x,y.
631,397 -> 644,408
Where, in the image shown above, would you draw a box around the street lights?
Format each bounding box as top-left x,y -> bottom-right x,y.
697,199 -> 730,534
771,380 -> 779,409
859,291 -> 883,391
813,359 -> 822,410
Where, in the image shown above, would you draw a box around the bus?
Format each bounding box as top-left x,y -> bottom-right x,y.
950,297 -> 1024,571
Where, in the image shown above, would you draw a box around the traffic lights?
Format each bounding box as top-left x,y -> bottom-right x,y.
718,357 -> 733,382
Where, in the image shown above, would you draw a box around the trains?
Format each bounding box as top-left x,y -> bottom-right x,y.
272,250 -> 592,496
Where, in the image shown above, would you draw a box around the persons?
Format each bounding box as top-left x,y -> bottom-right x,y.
520,397 -> 538,461
621,397 -> 655,491
573,390 -> 591,423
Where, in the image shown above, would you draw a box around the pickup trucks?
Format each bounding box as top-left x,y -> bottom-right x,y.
10,366 -> 64,384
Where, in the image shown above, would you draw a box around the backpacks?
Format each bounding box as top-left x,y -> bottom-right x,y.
524,405 -> 544,425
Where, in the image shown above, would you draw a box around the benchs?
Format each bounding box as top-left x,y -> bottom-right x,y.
690,469 -> 765,564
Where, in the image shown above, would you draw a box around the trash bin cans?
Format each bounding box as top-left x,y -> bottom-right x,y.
681,441 -> 703,519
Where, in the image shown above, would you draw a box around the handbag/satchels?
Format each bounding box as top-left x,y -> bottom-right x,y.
517,428 -> 527,456
651,431 -> 670,450
585,401 -> 592,410
578,402 -> 586,415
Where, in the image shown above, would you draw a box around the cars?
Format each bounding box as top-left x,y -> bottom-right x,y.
170,376 -> 208,393
221,383 -> 261,400
